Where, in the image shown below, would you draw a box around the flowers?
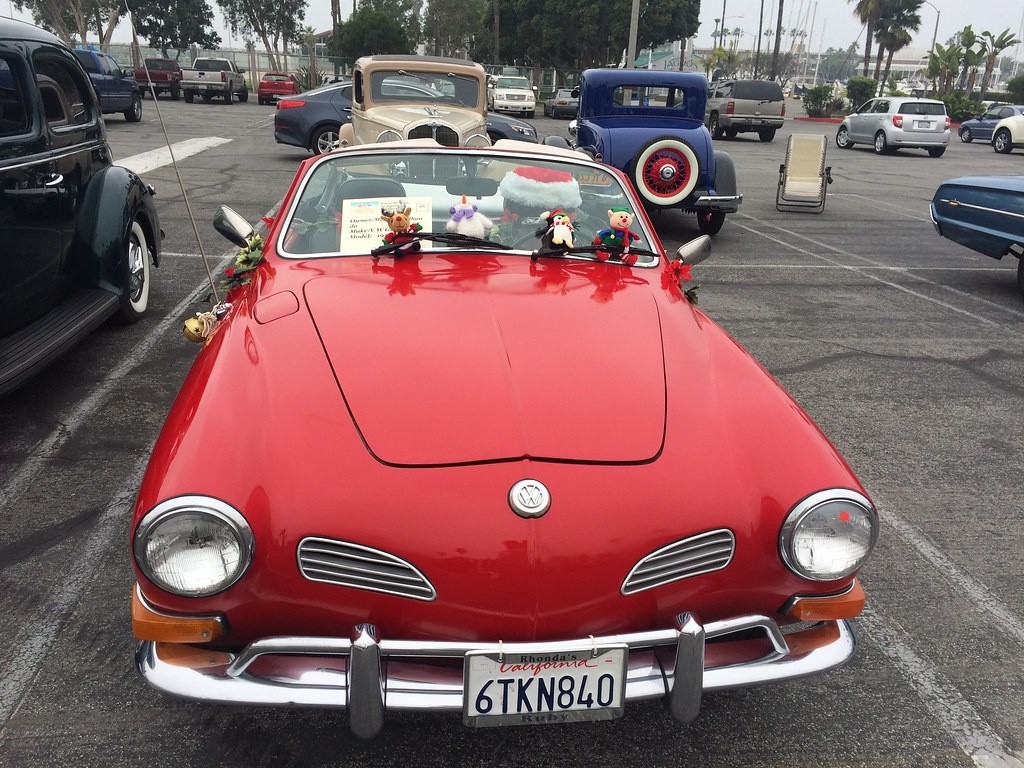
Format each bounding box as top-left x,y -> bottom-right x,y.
664,257 -> 701,304
191,210 -> 343,306
489,209 -> 576,245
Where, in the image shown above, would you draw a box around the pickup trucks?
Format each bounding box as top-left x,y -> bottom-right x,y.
1,49 -> 142,122
178,57 -> 249,104
136,58 -> 182,100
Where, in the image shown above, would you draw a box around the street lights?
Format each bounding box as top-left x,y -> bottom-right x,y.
714,16 -> 743,47
314,43 -> 326,82
922,0 -> 940,52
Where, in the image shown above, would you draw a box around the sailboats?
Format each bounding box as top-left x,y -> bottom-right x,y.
795,0 -> 826,94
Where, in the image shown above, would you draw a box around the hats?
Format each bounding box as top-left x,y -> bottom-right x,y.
497,165 -> 582,210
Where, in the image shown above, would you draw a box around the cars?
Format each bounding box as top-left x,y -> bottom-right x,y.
0,15 -> 165,397
258,73 -> 302,105
487,75 -> 538,119
130,146 -> 880,740
542,68 -> 743,235
991,111 -> 1024,154
929,175 -> 1024,296
836,97 -> 951,158
274,81 -> 538,155
958,105 -> 1024,143
338,54 -> 593,182
544,89 -> 579,119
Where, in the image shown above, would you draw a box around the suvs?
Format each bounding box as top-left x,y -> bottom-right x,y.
704,79 -> 785,142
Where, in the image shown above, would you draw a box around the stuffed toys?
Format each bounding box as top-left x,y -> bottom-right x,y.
375,201 -> 424,258
590,206 -> 643,266
538,207 -> 581,249
445,193 -> 495,242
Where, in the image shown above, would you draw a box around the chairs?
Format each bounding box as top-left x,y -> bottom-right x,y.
503,198 -> 596,251
306,177 -> 407,254
775,134 -> 833,213
